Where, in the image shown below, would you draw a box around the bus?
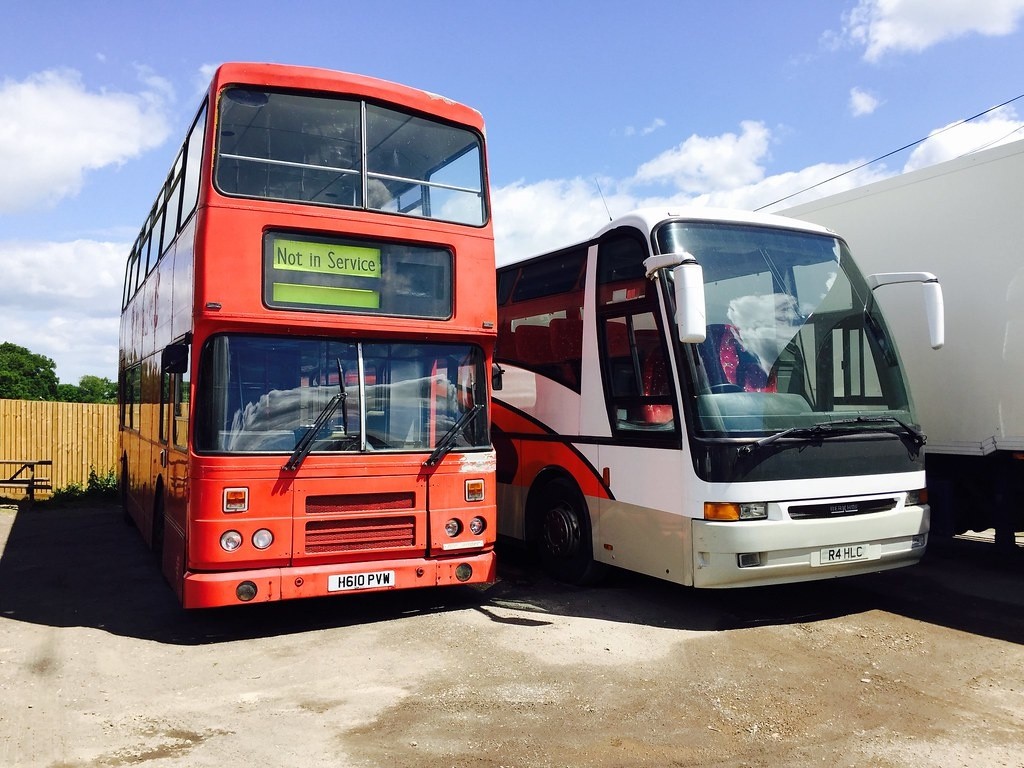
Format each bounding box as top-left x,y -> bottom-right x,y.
377,209 -> 952,591
111,60 -> 507,609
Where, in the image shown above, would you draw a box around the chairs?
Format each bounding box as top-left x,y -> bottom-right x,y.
293,370 -> 380,423
501,316 -> 777,425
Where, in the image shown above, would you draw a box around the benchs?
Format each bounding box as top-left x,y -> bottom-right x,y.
0,477 -> 51,489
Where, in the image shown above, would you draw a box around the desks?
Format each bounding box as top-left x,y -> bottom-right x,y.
0,460 -> 53,500
